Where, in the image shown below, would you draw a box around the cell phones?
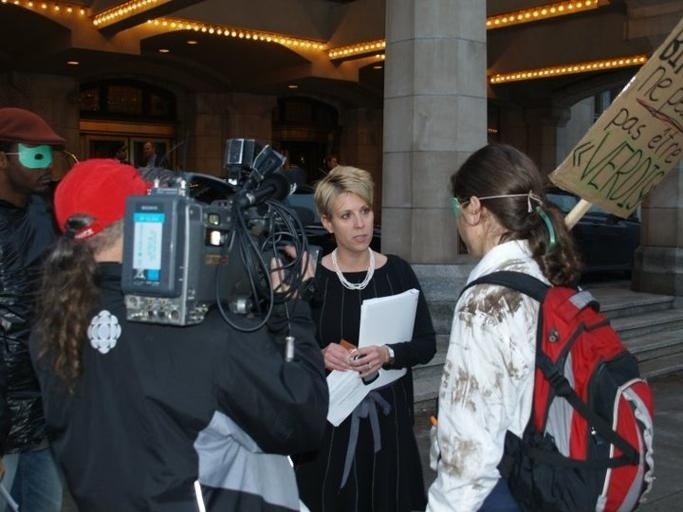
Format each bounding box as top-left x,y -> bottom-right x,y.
350,352 -> 380,387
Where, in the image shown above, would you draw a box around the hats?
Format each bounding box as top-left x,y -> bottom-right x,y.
55,159 -> 153,243
1,106 -> 66,145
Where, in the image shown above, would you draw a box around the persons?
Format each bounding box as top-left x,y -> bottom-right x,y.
0,106 -> 74,512
137,140 -> 175,169
26,155 -> 328,512
297,166 -> 437,512
105,139 -> 131,164
423,141 -> 585,512
311,154 -> 339,184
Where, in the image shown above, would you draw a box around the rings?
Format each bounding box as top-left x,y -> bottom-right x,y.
366,363 -> 373,370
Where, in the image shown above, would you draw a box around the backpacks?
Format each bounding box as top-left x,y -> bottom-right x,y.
457,271 -> 655,512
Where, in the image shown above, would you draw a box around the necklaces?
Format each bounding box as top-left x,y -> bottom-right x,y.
330,245 -> 375,291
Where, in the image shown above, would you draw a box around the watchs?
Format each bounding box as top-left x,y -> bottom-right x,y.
381,344 -> 395,365
